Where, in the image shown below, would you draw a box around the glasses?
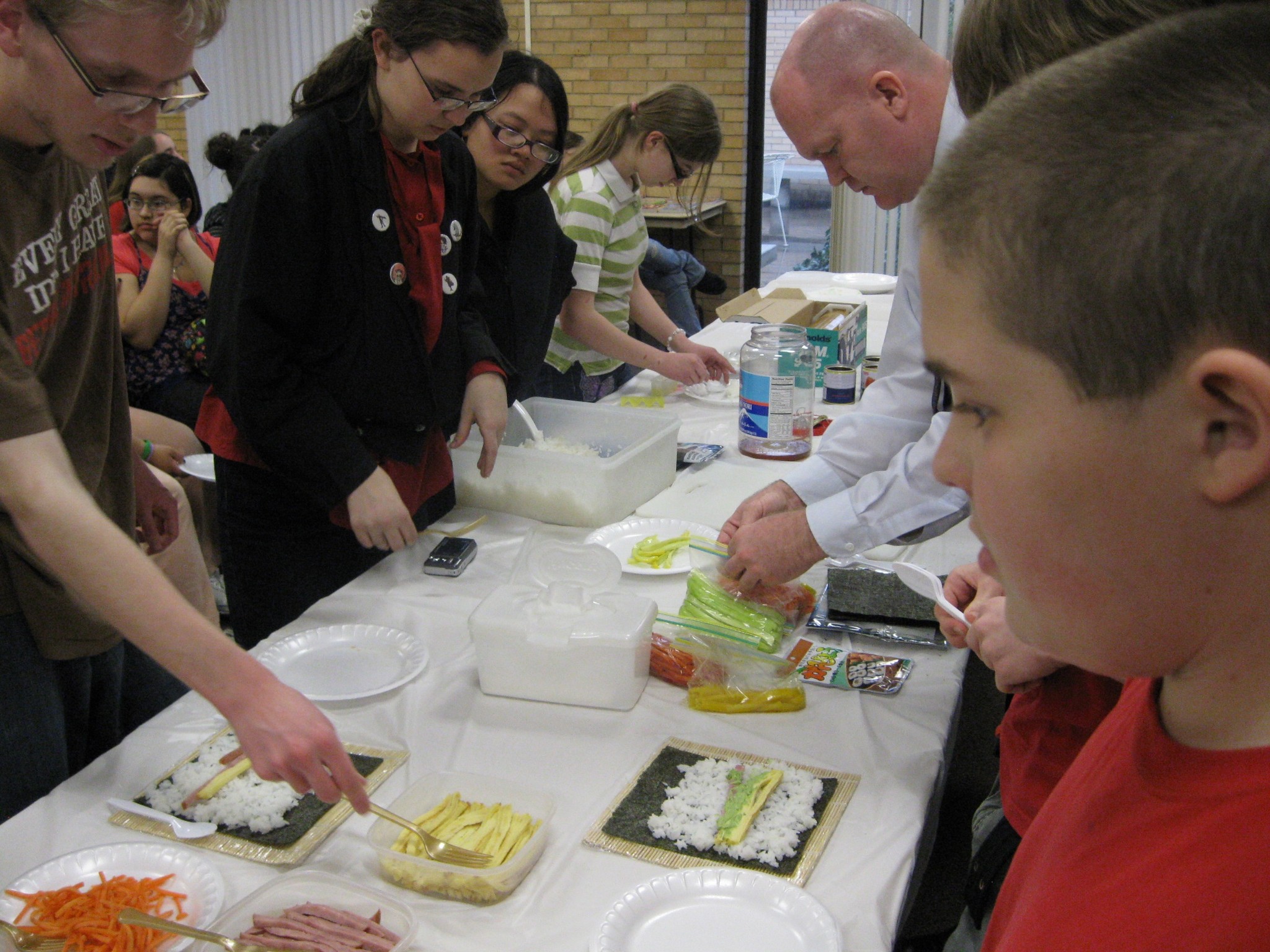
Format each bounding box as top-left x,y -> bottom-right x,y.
647,131 -> 690,180
404,45 -> 498,112
124,196 -> 186,210
480,110 -> 563,165
24,0 -> 211,114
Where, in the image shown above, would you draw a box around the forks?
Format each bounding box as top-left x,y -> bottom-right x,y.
341,791 -> 494,870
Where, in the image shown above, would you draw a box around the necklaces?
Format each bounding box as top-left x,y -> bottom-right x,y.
173,258 -> 185,272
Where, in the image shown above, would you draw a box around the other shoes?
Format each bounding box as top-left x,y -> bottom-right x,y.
209,574 -> 230,612
694,270 -> 727,295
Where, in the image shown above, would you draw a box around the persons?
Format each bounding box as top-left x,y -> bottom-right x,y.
913,0 -> 1270,952
557,130 -> 727,338
446,48 -> 578,404
542,84 -> 736,405
100,123 -> 279,620
0,0 -> 370,817
933,0 -> 1216,952
206,0 -> 519,652
714,1 -> 972,594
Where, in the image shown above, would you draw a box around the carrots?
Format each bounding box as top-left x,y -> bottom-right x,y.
4,871 -> 188,952
718,574 -> 816,617
650,632 -> 731,689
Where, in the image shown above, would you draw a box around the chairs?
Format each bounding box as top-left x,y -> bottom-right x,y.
763,153 -> 797,246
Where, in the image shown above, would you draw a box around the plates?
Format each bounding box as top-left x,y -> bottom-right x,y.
0,841 -> 227,952
684,378 -> 740,406
191,867 -> 423,952
833,272 -> 897,295
584,518 -> 719,574
804,566 -> 949,649
596,865 -> 845,952
255,623 -> 430,701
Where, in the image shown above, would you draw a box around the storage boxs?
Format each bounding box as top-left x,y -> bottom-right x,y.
715,286 -> 867,389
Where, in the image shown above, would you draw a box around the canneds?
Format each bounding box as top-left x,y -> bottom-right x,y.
821,355 -> 880,405
740,323 -> 816,460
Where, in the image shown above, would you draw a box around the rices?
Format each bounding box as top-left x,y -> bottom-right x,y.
143,733 -> 305,834
646,755 -> 825,868
515,436 -> 601,459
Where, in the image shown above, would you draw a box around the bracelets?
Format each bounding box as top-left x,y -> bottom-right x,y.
142,440 -> 151,460
666,328 -> 687,352
148,444 -> 155,462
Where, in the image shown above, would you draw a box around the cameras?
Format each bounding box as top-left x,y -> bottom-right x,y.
422,536 -> 478,577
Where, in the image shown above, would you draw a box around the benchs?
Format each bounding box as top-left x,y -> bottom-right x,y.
766,163 -> 828,208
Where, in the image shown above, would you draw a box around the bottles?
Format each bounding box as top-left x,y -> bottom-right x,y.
737,322 -> 817,460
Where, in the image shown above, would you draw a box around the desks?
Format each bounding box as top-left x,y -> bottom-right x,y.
641,196 -> 727,329
0,268 -> 990,952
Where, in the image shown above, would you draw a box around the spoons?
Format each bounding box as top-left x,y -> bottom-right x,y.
108,797 -> 216,840
891,560 -> 974,630
825,555 -> 895,571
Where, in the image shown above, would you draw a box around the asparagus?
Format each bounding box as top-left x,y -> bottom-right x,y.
730,771 -> 784,841
684,683 -> 806,713
385,792 -> 544,902
678,572 -> 786,654
198,756 -> 251,798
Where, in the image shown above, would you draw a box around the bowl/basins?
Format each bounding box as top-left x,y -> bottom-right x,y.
468,580 -> 662,710
360,773 -> 560,907
